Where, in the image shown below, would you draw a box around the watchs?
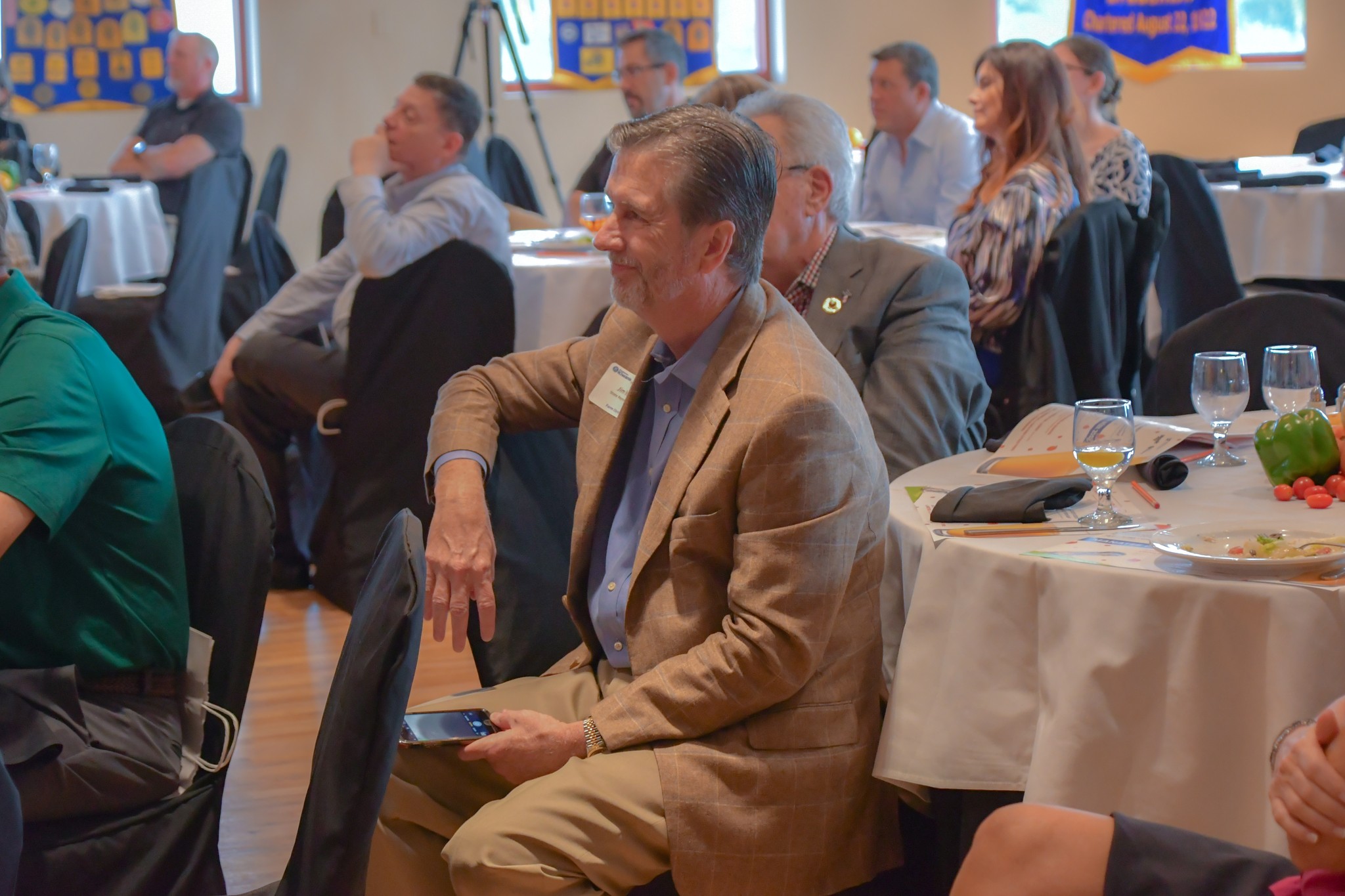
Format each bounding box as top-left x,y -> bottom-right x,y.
579,716 -> 607,759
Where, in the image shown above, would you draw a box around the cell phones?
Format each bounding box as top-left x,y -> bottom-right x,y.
397,707 -> 496,748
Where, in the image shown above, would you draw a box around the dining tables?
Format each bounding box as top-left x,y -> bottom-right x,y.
1190,151 -> 1344,305
877,409 -> 1344,867
0,173 -> 181,297
484,219 -> 962,352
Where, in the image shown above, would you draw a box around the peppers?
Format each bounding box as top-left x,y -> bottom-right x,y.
1253,406 -> 1341,487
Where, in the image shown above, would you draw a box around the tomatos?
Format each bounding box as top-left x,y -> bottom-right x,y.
1274,474 -> 1345,509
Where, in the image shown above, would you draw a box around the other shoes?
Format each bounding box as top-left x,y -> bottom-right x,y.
266,549 -> 314,588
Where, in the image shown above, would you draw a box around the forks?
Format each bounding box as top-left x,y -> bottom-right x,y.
1253,532 -> 1345,551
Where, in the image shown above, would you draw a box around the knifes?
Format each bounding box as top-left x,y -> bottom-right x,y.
965,524 -> 1142,536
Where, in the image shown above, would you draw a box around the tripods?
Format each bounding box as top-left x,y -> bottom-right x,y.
450,0 -> 569,212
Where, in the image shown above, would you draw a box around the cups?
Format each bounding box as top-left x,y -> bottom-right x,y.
579,190 -> 612,232
1262,341 -> 1320,420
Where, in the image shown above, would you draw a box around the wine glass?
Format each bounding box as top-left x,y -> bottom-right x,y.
1192,351 -> 1253,467
1071,394 -> 1139,531
32,143 -> 59,190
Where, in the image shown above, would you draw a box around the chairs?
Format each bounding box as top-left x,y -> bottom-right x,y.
969,174 -> 1173,440
73,147 -> 298,403
0,414 -> 694,896
290,237 -> 520,619
1146,289 -> 1344,414
317,135 -> 542,256
1148,151 -> 1255,375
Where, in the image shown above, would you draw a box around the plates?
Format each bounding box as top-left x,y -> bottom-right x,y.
1152,516 -> 1345,577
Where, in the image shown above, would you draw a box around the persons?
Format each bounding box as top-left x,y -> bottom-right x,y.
350,102 -> 896,895
945,692 -> 1345,895
731,82 -> 994,478
934,36 -> 1084,403
102,30 -> 243,261
1046,30 -> 1154,245
565,27 -> 687,239
846,38 -> 986,242
209,72 -> 514,586
0,249 -> 209,826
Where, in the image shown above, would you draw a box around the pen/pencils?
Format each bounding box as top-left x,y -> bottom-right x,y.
1130,480 -> 1160,508
1179,445 -> 1229,462
